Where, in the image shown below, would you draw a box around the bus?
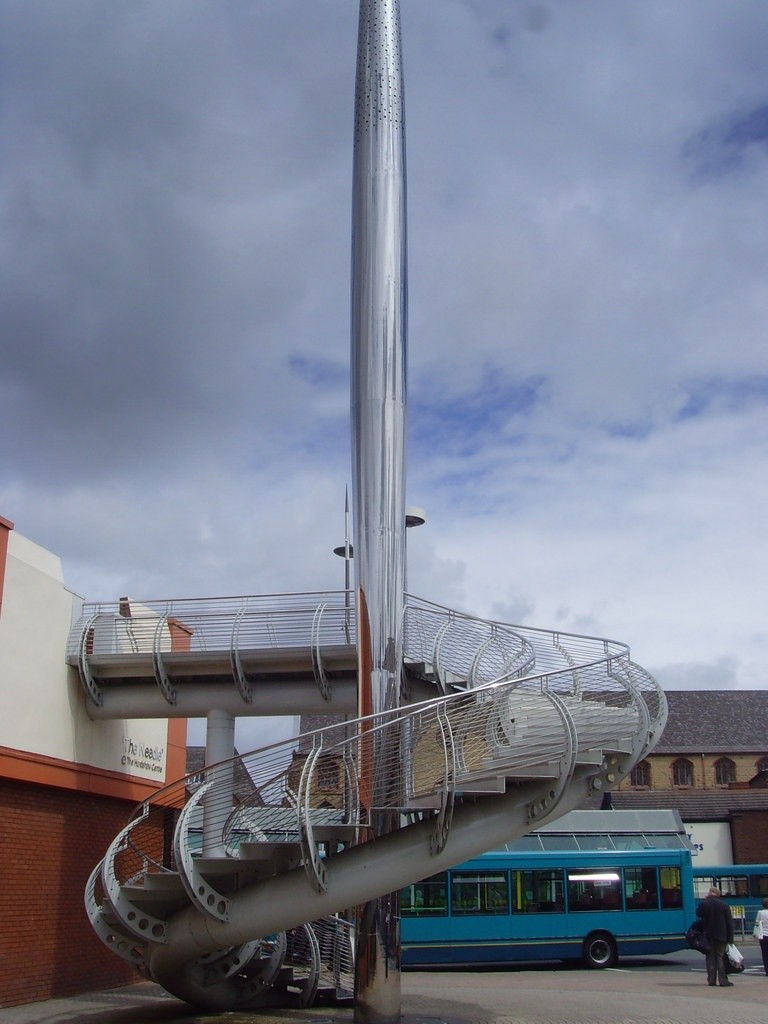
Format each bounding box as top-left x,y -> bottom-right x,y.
625,863 -> 768,935
183,804 -> 698,969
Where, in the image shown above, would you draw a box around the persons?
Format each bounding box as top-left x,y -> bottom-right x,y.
577,886 -> 655,910
696,886 -> 736,987
753,898 -> 768,976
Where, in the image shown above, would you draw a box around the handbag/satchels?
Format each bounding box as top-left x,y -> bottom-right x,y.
726,944 -> 744,969
752,911 -> 764,940
687,922 -> 711,954
723,946 -> 745,974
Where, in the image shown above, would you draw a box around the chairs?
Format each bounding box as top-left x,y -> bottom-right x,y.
576,889 -> 681,909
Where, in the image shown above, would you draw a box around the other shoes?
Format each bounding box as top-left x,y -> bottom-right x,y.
720,983 -> 733,987
709,983 -> 716,986
